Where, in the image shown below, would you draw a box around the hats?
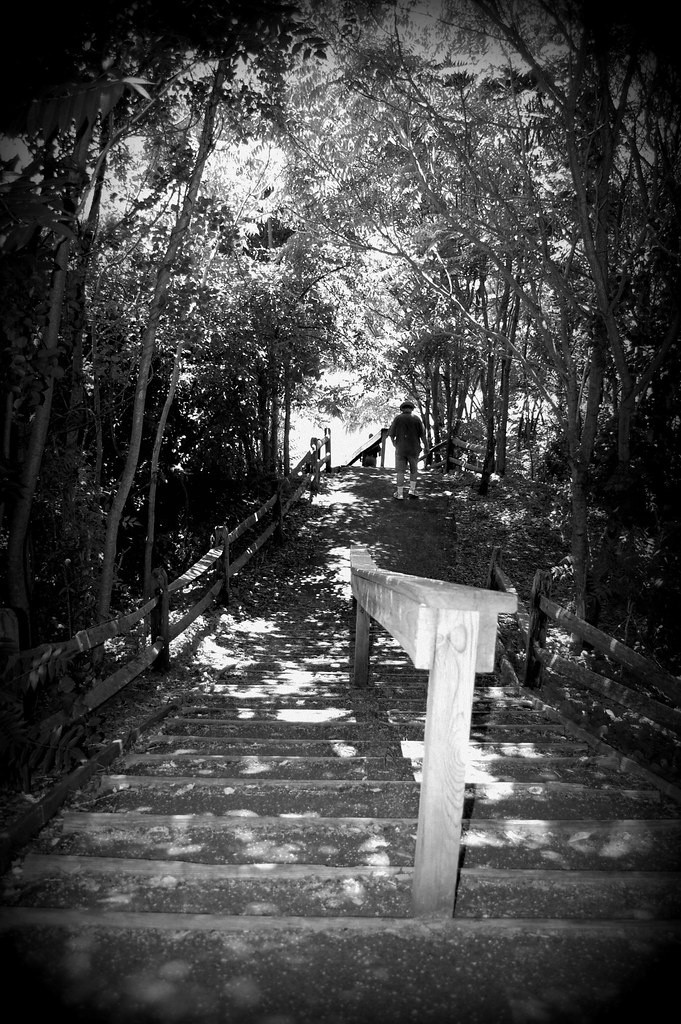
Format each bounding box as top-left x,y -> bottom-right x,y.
400,402 -> 415,410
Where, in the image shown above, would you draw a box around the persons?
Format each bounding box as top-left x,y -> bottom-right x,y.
359,433 -> 381,468
386,402 -> 430,501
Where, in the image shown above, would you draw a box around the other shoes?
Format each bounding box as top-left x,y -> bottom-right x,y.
408,491 -> 419,498
393,492 -> 403,499
